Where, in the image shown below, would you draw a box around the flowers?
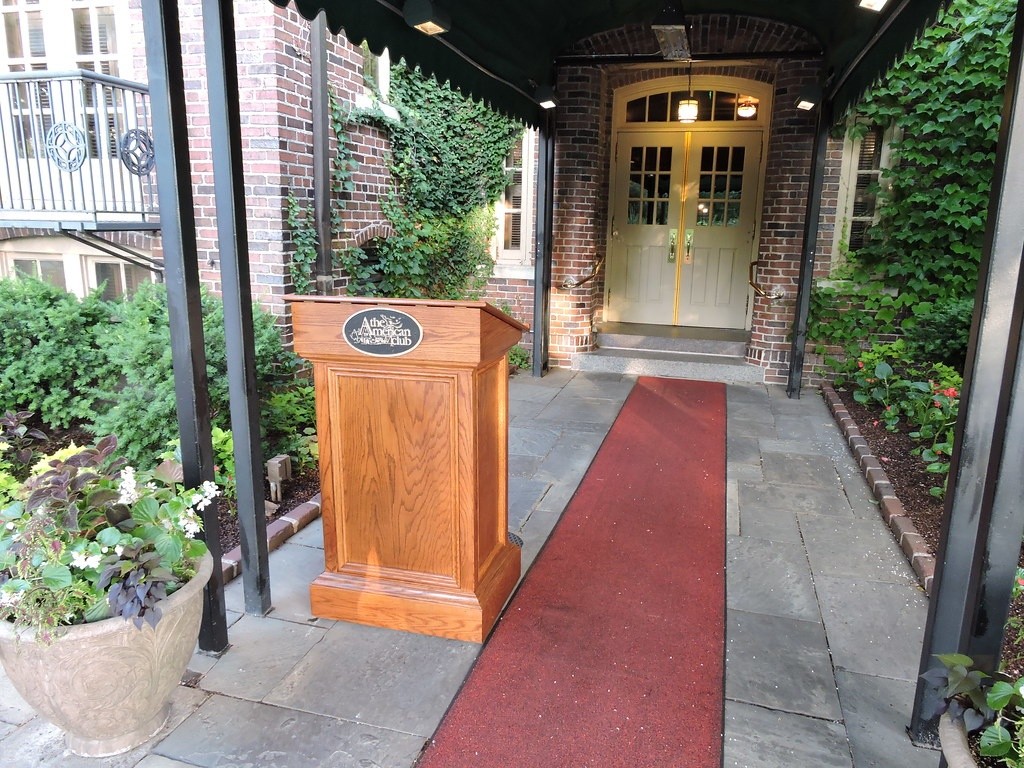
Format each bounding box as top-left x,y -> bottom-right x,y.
0,432 -> 221,646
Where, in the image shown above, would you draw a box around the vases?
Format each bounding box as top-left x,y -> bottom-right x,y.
0,549 -> 214,758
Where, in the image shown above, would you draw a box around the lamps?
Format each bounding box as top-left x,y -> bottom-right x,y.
403,0 -> 451,36
535,86 -> 560,109
678,62 -> 698,124
794,87 -> 821,112
855,0 -> 890,15
